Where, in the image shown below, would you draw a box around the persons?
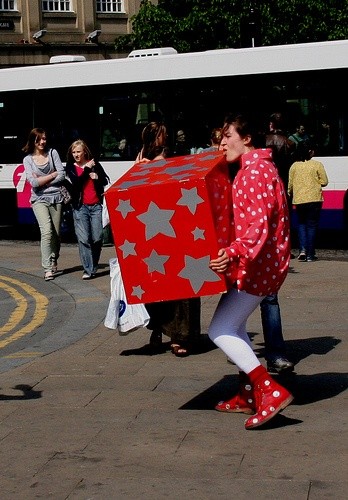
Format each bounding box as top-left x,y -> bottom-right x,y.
134,113 -> 328,357
23,128 -> 65,281
104,107 -> 348,158
66,140 -> 110,279
208,113 -> 291,428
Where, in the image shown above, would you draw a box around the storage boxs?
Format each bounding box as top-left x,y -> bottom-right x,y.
103,150 -> 239,305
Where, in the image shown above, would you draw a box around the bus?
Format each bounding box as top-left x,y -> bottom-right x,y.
0,38 -> 348,233
